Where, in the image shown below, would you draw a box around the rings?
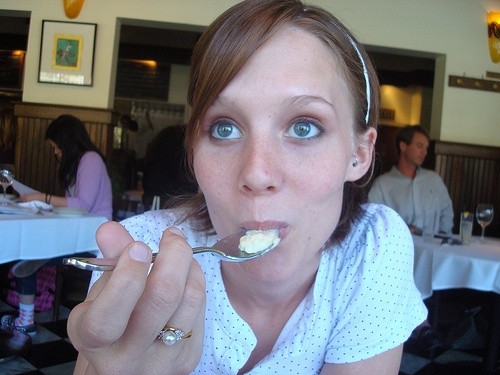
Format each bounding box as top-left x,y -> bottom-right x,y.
155,326 -> 192,344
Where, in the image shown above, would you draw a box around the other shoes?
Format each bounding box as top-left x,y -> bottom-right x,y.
12,258 -> 53,277
415,325 -> 444,353
0,315 -> 38,337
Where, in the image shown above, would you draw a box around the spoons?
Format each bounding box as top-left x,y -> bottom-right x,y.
63,230 -> 281,271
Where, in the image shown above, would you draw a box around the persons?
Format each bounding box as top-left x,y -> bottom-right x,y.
64,1 -> 430,374
0,113 -> 114,333
141,123 -> 200,213
366,123 -> 456,358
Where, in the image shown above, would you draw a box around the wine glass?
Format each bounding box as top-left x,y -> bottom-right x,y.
476,204 -> 494,244
0,170 -> 15,206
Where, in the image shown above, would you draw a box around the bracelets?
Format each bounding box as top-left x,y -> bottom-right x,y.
48,193 -> 51,204
45,192 -> 47,203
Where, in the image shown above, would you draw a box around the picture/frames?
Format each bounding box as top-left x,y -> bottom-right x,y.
38,20 -> 98,87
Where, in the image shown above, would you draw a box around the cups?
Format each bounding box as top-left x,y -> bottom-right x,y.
459,212 -> 474,245
422,209 -> 437,244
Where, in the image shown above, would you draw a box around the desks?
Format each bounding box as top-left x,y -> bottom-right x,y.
0,207 -> 109,322
414,234 -> 500,367
